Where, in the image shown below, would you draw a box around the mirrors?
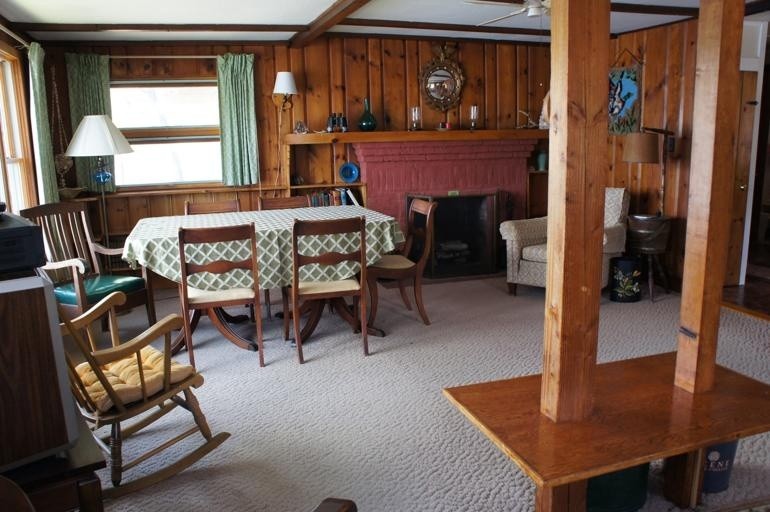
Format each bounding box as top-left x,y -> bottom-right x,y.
418,50 -> 470,113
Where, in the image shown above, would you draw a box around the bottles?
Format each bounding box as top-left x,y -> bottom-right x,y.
537,148 -> 548,171
323,112 -> 347,133
358,97 -> 377,132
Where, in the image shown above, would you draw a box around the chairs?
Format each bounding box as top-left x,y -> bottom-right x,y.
18,194 -> 157,331
175,226 -> 267,371
499,187 -> 630,301
280,216 -> 368,365
49,270 -> 230,500
368,193 -> 438,324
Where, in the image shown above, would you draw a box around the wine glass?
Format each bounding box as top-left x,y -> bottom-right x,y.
468,105 -> 480,130
410,106 -> 420,132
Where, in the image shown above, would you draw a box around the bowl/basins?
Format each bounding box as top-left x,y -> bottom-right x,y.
57,186 -> 88,200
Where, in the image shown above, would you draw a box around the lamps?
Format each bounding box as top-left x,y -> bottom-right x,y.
617,125 -> 672,309
271,69 -> 301,110
62,114 -> 136,274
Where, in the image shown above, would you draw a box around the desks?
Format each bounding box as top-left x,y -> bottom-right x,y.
281,128 -> 563,288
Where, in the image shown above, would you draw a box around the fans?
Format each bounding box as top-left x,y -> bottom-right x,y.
460,0 -> 556,31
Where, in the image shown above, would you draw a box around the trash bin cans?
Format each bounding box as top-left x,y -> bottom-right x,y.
609,256 -> 644,303
